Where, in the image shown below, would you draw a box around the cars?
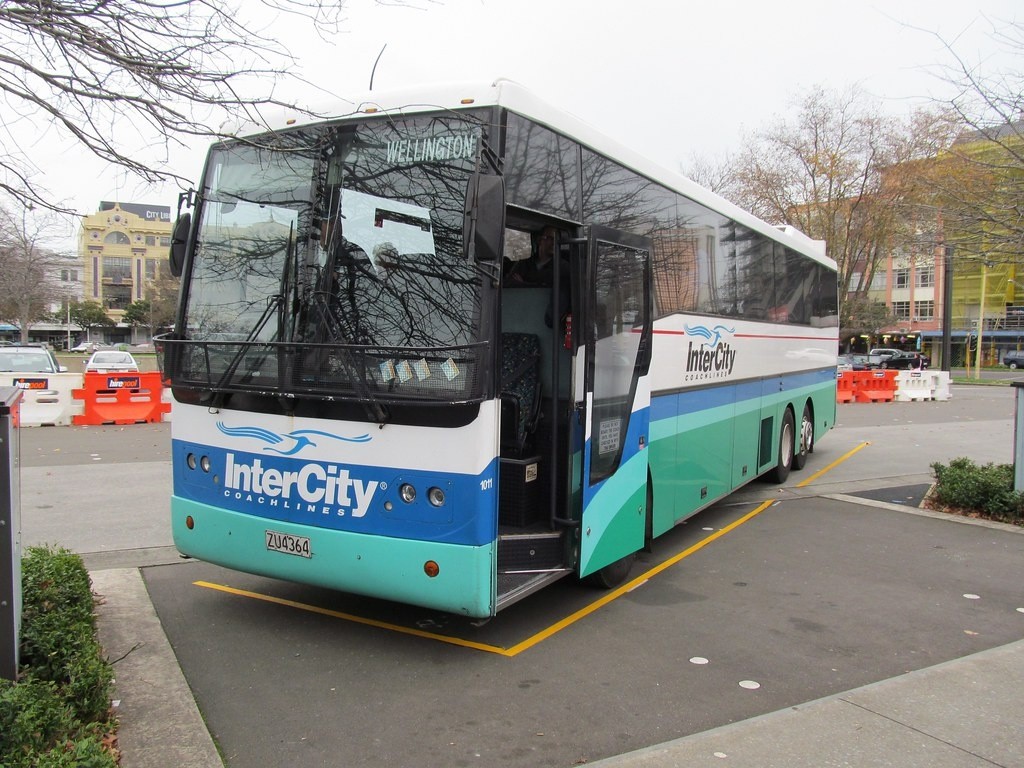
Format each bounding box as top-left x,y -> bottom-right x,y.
877,351 -> 931,370
839,356 -> 872,372
870,348 -> 903,358
82,350 -> 143,393
1002,348 -> 1024,369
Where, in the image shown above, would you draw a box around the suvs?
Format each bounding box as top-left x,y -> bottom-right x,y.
0,339 -> 69,403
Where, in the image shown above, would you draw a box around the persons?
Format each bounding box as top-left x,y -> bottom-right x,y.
506,224 -> 570,288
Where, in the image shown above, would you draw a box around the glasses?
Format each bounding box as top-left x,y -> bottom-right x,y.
539,235 -> 553,241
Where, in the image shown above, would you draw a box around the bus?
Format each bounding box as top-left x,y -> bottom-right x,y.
150,75 -> 840,626
